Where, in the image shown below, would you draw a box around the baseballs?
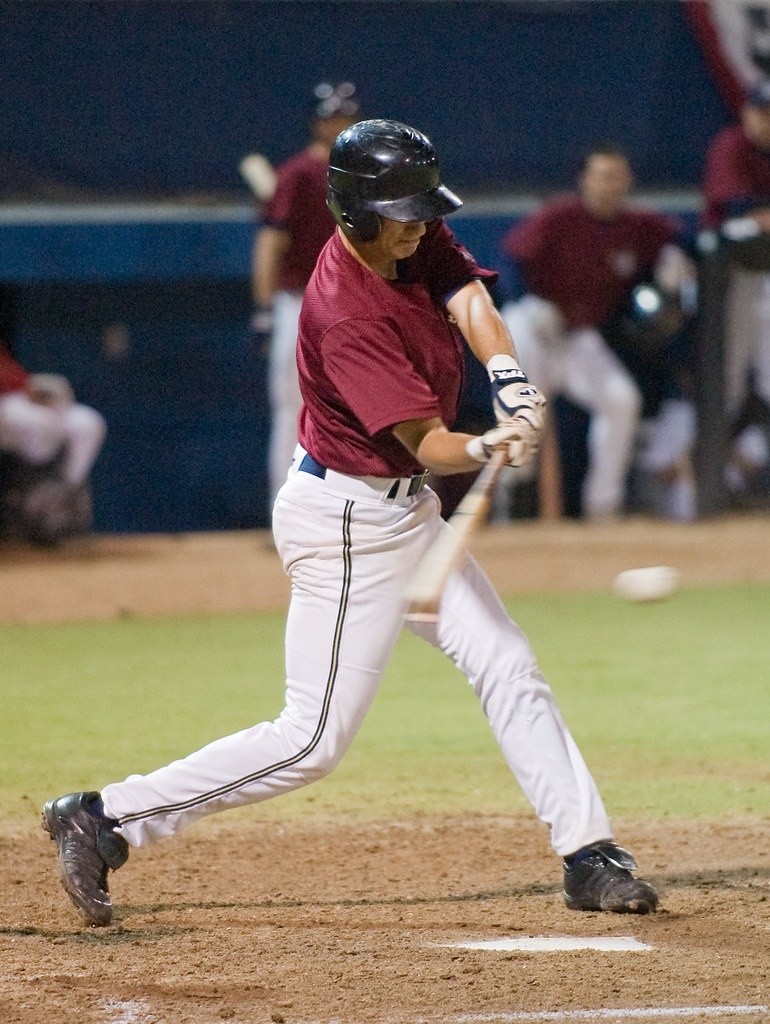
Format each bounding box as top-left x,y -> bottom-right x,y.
613,563 -> 681,603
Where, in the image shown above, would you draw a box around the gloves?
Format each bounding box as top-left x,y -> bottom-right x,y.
482,369 -> 547,468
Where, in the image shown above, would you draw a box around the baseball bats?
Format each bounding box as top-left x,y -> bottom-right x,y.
401,449 -> 508,625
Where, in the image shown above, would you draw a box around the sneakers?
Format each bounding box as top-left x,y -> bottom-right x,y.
42,791 -> 128,926
564,842 -> 658,913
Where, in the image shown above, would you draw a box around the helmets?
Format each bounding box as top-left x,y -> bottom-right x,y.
325,120 -> 465,245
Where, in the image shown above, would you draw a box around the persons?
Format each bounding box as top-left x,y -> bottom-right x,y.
0,345 -> 106,543
700,77 -> 770,505
492,151 -> 698,518
243,72 -> 365,539
39,117 -> 665,923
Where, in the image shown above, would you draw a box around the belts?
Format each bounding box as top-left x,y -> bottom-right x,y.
386,477 -> 424,499
